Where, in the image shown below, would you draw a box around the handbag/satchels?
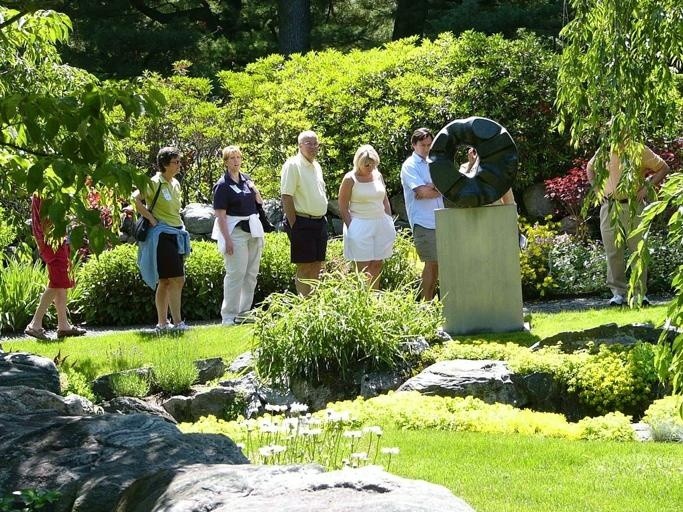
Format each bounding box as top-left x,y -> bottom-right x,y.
134,216 -> 149,241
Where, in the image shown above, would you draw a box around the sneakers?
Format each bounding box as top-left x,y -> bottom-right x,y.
234,311 -> 256,323
609,294 -> 627,305
24,325 -> 50,340
171,321 -> 189,331
156,320 -> 173,332
643,296 -> 650,305
57,325 -> 86,337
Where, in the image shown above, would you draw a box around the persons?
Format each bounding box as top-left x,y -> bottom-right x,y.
337,146 -> 396,291
280,130 -> 329,300
459,148 -> 515,205
24,195 -> 86,340
212,145 -> 264,325
400,127 -> 444,303
586,119 -> 670,306
131,147 -> 195,331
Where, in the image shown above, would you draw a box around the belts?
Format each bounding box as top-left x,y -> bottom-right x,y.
608,198 -> 628,203
296,212 -> 323,219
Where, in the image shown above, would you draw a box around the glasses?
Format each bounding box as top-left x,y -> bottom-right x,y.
169,161 -> 179,164
